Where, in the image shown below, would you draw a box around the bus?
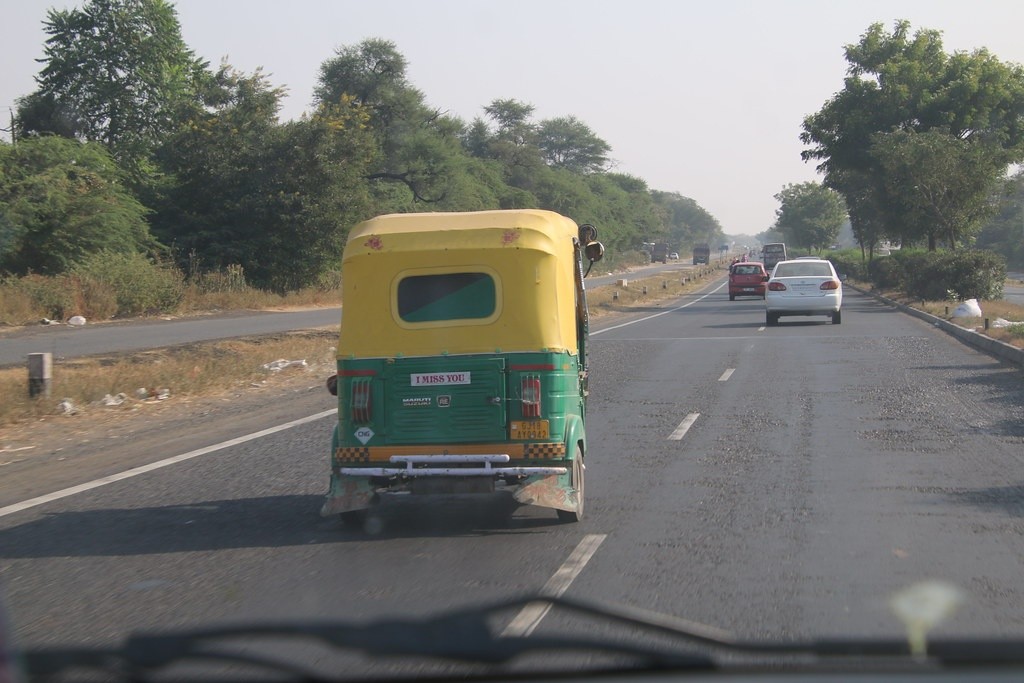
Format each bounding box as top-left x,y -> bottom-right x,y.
761,243 -> 786,270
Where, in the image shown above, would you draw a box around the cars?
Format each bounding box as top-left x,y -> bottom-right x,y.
765,259 -> 842,326
670,253 -> 679,260
729,262 -> 768,301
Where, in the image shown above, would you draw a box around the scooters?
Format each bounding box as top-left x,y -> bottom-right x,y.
320,209 -> 604,528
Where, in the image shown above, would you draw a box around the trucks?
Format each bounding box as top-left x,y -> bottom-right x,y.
692,242 -> 711,265
